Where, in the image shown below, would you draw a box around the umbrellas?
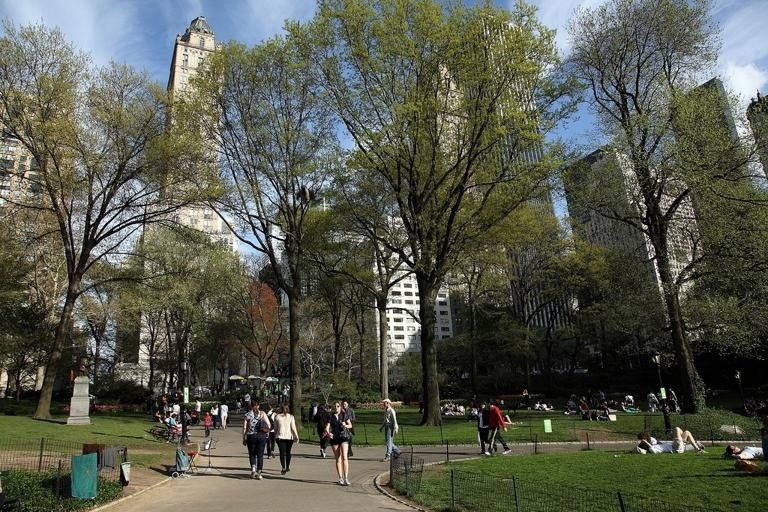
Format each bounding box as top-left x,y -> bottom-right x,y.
262,376 -> 280,384
245,374 -> 260,384
226,374 -> 244,386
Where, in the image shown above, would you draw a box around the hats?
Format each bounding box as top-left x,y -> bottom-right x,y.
382,399 -> 391,404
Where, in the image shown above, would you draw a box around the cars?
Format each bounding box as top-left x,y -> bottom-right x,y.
0,382 -> 34,399
164,384 -> 211,398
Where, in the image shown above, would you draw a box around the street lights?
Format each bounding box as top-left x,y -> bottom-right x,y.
648,351 -> 672,435
732,369 -> 747,416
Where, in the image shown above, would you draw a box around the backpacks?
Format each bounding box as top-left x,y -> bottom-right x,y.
266,412 -> 274,431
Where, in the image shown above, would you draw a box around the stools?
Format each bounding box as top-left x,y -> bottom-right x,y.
187,450 -> 198,473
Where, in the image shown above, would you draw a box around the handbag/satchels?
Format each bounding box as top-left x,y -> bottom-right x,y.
255,418 -> 270,433
341,425 -> 354,441
477,431 -> 481,447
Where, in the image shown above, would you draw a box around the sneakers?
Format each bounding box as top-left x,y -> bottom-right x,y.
494,444 -> 497,452
319,449 -> 326,459
281,469 -> 286,475
287,468 -> 290,472
382,450 -> 403,461
478,451 -> 485,454
271,450 -> 275,459
268,455 -> 273,459
344,479 -> 350,486
250,472 -> 256,479
338,478 -> 344,485
256,474 -> 263,480
502,449 -> 512,454
484,452 -> 492,456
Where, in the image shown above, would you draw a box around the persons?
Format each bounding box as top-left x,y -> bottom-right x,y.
378,398 -> 402,461
242,400 -> 271,480
723,437 -> 768,459
308,400 -> 319,435
315,405 -> 331,458
153,383 -> 290,443
639,432 -> 705,451
321,400 -> 353,485
638,427 -> 709,454
419,388 -> 680,425
342,401 -> 356,458
484,398 -> 512,455
476,400 -> 497,454
275,404 -> 300,474
266,400 -> 277,458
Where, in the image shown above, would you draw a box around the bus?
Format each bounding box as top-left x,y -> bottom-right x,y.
498,394 -> 546,407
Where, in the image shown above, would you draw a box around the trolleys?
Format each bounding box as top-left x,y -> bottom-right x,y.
171,434 -> 193,480
